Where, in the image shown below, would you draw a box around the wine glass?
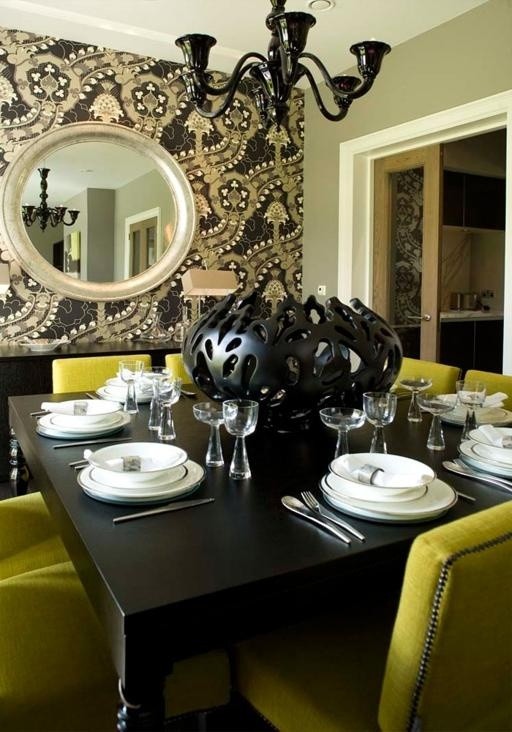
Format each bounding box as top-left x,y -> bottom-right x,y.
192,399 -> 260,481
118,359 -> 183,440
319,373 -> 486,459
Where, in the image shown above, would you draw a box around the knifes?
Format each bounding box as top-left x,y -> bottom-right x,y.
112,498 -> 216,524
52,436 -> 132,448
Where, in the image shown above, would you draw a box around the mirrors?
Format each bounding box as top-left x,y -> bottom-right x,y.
2,120 -> 196,303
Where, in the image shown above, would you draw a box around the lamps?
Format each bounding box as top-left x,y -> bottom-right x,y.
174,0 -> 393,133
21,168 -> 80,233
181,268 -> 238,320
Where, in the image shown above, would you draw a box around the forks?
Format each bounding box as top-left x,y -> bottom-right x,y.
300,491 -> 368,541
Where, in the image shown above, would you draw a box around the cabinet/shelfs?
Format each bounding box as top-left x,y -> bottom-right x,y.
394,320 -> 503,379
443,169 -> 506,231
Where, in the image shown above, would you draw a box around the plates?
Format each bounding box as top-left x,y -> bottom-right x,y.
315,452 -> 458,522
428,394 -> 512,476
35,374 -> 168,439
77,442 -> 207,504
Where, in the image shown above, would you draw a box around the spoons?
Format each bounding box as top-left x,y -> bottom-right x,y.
442,457 -> 512,492
280,495 -> 351,544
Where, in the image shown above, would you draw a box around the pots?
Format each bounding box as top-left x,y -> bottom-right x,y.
450,292 -> 481,310
